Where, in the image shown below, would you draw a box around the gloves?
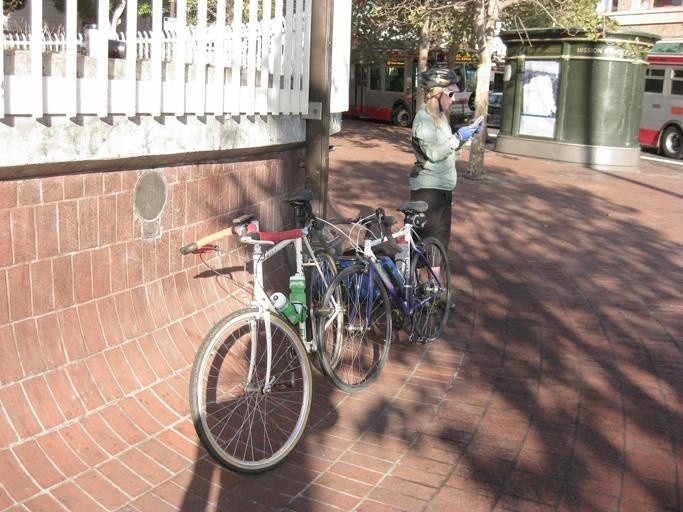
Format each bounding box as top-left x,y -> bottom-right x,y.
456,120 -> 483,141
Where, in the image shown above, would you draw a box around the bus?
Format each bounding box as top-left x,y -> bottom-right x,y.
634,51 -> 682,161
341,42 -> 505,129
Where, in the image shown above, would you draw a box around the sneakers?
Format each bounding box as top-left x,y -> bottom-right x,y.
416,292 -> 456,315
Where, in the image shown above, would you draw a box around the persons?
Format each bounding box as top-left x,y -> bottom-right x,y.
408,65 -> 483,314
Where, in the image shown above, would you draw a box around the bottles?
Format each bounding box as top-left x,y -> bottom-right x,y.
380,241 -> 408,289
270,272 -> 306,326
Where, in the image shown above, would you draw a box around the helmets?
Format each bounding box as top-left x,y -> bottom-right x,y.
421,67 -> 458,95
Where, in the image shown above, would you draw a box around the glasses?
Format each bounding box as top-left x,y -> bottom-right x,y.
444,91 -> 455,98
